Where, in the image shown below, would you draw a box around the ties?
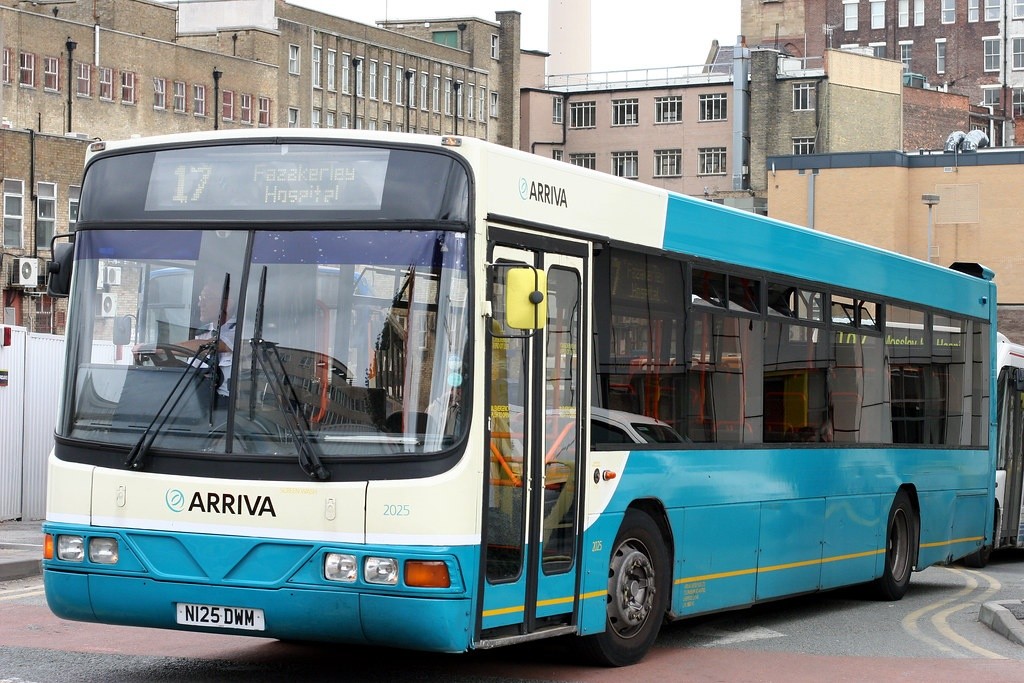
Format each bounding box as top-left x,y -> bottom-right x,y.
208,330 -> 219,368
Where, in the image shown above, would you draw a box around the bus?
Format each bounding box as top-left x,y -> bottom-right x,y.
40,126 -> 1003,666
811,317 -> 1024,567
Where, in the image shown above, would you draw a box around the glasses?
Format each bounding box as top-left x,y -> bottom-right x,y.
199,296 -> 211,304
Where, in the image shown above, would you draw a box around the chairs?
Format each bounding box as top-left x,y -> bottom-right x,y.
661,416 -> 826,443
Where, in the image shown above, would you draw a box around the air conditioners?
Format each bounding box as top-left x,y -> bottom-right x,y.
104,267 -> 122,286
94,292 -> 118,320
11,258 -> 39,288
64,132 -> 89,140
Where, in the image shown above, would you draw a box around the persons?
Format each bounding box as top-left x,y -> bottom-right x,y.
131,281 -> 245,410
